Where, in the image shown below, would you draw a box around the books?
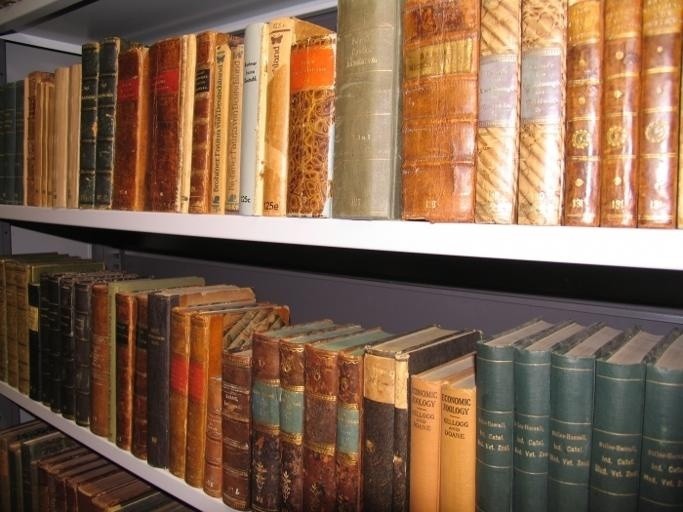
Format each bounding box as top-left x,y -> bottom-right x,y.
1,15 -> 336,218
333,0 -> 683,231
0,252 -> 292,512
250,316 -> 683,512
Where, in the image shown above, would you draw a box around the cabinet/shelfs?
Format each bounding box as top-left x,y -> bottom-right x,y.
1,2 -> 683,512
